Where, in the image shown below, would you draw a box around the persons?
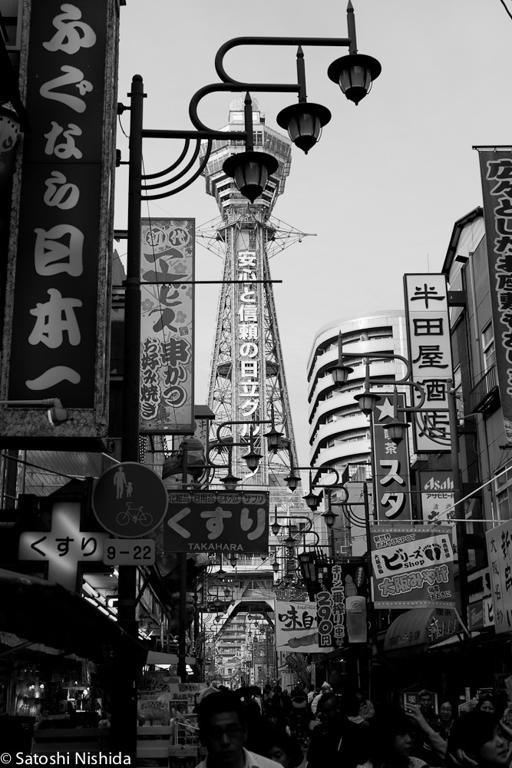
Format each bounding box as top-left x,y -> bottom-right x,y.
196,678 -> 509,765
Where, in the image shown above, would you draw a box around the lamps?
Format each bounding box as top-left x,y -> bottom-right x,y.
206,550 -> 237,623
246,613 -> 265,642
264,463 -> 349,573
330,351 -> 425,447
201,416 -> 283,491
189,37 -> 381,206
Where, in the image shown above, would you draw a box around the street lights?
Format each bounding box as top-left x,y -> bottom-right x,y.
321,324 -> 480,692
107,0 -> 390,767
170,388 -> 380,748
